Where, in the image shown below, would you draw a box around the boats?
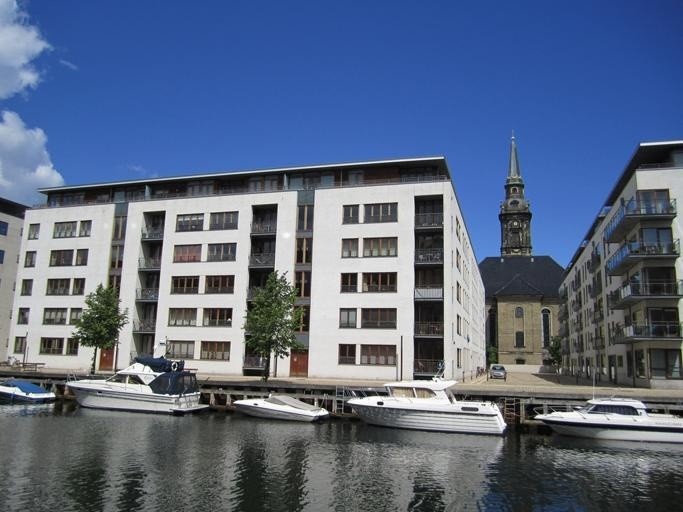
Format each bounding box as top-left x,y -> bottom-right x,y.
0,379 -> 56,401
66,356 -> 210,414
533,395 -> 683,443
232,395 -> 328,422
345,379 -> 506,435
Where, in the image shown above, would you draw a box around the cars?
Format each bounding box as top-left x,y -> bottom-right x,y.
490,365 -> 507,379
